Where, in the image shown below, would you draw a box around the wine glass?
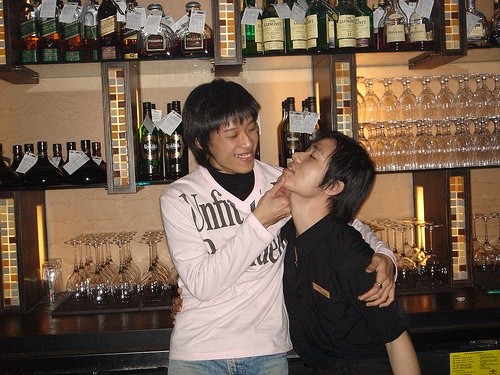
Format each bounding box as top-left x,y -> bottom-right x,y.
355,72 -> 500,173
360,216 -> 448,290
471,212 -> 500,273
64,230 -> 180,306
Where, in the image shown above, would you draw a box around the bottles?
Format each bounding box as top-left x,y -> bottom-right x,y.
0,139 -> 107,190
277,96 -> 321,167
465,0 -> 490,48
18,0 -> 214,66
139,100 -> 189,181
239,0 -> 437,58
488,0 -> 500,47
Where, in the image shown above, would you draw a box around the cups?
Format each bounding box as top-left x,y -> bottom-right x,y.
42,257 -> 62,303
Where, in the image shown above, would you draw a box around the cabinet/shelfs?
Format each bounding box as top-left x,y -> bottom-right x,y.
1,0 -> 500,375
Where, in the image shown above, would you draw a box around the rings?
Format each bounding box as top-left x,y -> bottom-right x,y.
375,282 -> 382,288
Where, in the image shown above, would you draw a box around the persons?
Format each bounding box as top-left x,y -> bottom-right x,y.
160,79 -> 422,375
